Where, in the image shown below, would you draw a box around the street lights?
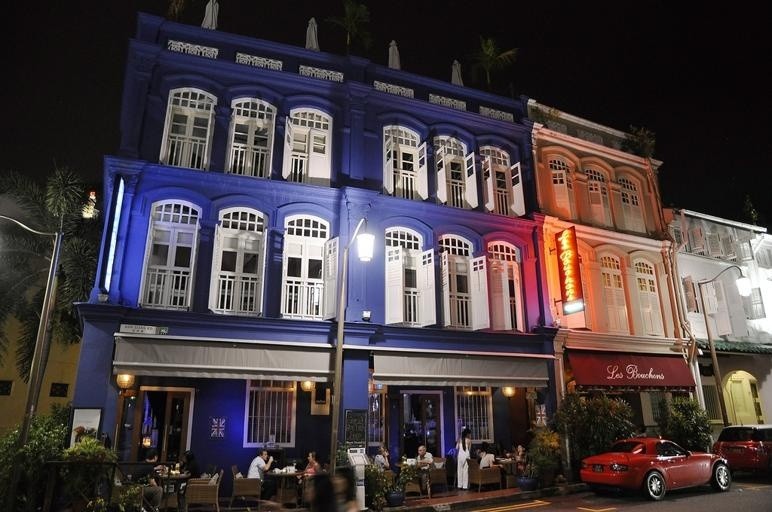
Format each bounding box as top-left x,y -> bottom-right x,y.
698,266 -> 755,429
324,213 -> 383,482
12,183 -> 100,469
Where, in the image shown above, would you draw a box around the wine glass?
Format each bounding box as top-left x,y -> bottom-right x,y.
263,456 -> 278,465
282,459 -> 298,472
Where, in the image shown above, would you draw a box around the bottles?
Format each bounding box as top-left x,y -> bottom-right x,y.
400,454 -> 416,465
162,463 -> 181,476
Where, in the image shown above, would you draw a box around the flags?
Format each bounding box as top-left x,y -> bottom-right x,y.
208,415 -> 225,440
535,403 -> 548,425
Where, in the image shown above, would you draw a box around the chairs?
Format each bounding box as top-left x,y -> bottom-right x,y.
115,464 -> 333,512
376,449 -> 527,494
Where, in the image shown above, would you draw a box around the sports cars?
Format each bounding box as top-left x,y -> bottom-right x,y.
577,438 -> 737,501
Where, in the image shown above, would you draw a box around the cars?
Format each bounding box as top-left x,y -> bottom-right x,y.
712,423 -> 772,482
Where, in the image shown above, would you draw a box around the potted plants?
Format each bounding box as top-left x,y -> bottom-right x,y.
382,454 -> 419,507
517,443 -> 552,491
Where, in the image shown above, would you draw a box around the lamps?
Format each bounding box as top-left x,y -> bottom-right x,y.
115,372 -> 136,390
501,386 -> 516,400
300,380 -> 316,392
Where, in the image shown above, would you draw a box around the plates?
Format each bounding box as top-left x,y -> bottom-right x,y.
497,453 -> 515,462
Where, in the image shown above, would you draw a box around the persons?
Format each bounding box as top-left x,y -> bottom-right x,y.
295,450 -> 324,508
174,450 -> 200,511
248,449 -> 277,498
456,428 -> 472,490
131,448 -> 163,512
472,439 -> 528,488
414,445 -> 434,495
372,444 -> 391,472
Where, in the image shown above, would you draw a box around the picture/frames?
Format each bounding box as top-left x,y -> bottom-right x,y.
344,408 -> 369,450
68,407 -> 104,449
